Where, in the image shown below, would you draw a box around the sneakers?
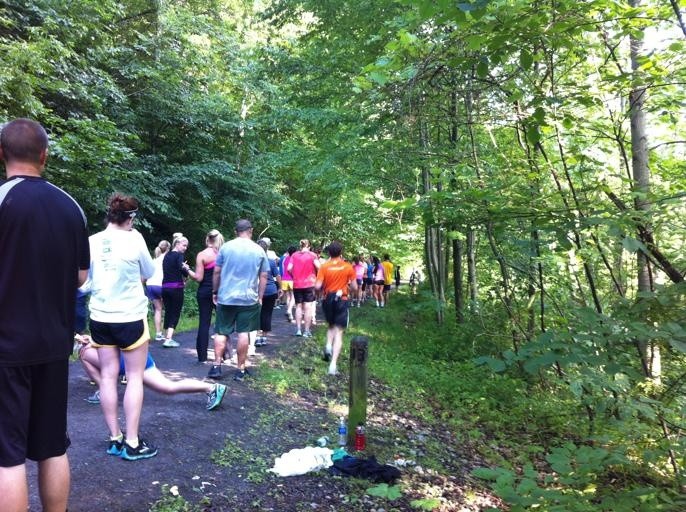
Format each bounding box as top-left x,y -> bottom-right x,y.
118,440 -> 159,461
321,348 -> 331,362
349,301 -> 355,306
357,303 -> 361,307
327,365 -> 339,377
380,301 -> 384,307
302,330 -> 313,338
254,336 -> 269,346
106,433 -> 124,458
154,330 -> 164,341
120,375 -> 126,387
296,328 -> 302,338
89,381 -> 96,386
83,390 -> 101,405
280,302 -> 287,307
207,363 -> 224,378
232,369 -> 250,381
376,301 -> 379,308
205,382 -> 227,411
162,336 -> 182,349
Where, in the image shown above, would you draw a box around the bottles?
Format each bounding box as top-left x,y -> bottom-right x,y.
338,415 -> 348,445
354,422 -> 366,450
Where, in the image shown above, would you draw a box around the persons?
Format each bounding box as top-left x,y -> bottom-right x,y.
347,251 -> 374,310
282,246 -> 296,323
75,193 -> 160,462
160,231 -> 189,348
140,239 -> 172,342
381,253 -> 394,309
278,250 -> 287,306
180,228 -> 232,366
207,219 -> 271,383
261,236 -> 282,310
286,239 -> 321,338
394,265 -> 401,295
314,241 -> 359,377
408,270 -> 424,290
371,256 -> 386,308
0,117 -> 91,512
255,239 -> 284,348
246,328 -> 258,357
77,273 -> 231,412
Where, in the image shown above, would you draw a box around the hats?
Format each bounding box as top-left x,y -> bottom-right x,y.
235,218 -> 252,232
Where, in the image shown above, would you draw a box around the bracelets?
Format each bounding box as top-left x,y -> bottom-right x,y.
186,268 -> 192,273
